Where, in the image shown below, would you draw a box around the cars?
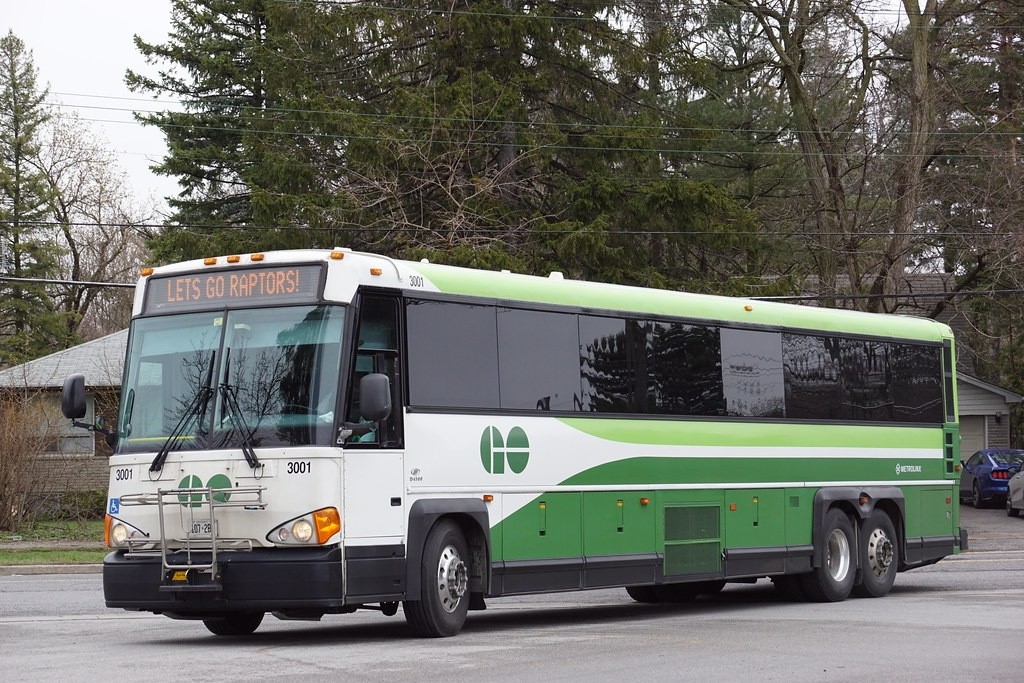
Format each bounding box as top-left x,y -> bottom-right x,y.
1005,461 -> 1024,517
959,449 -> 1024,510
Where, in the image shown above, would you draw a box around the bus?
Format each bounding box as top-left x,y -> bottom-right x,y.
59,248 -> 968,640
